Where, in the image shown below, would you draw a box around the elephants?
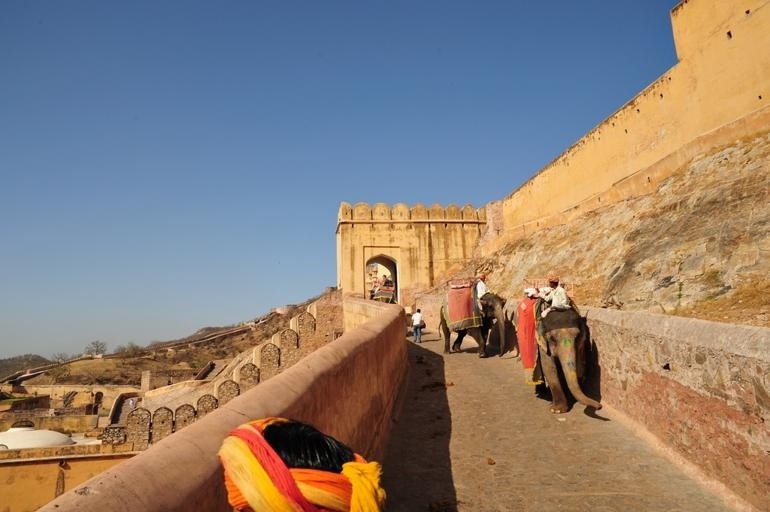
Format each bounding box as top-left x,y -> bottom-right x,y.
513,294 -> 603,414
438,292 -> 507,358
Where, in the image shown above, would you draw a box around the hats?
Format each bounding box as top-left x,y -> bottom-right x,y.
549,276 -> 558,283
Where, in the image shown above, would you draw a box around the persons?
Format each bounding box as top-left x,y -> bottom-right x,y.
410,309 -> 422,343
477,274 -> 489,309
215,417 -> 388,511
539,276 -> 571,318
382,274 -> 393,286
